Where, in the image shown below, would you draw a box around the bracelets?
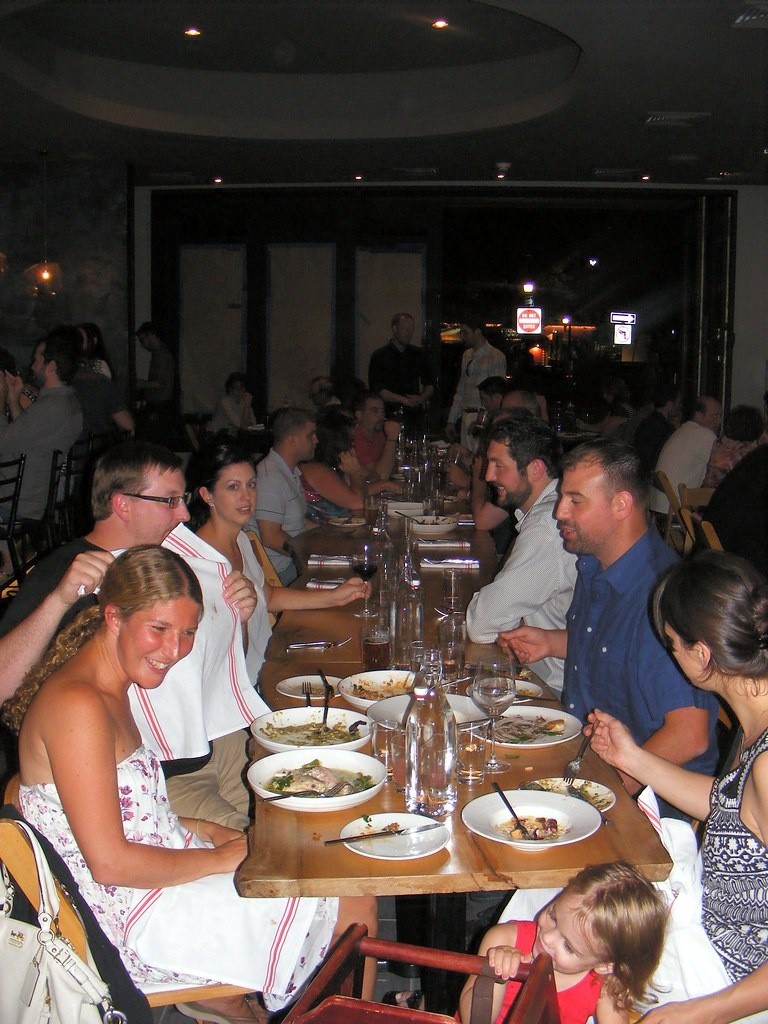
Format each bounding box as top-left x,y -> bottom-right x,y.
196,818 -> 205,834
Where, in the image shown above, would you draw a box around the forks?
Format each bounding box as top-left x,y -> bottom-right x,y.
302,682 -> 312,708
263,781 -> 346,802
563,717 -> 600,786
492,781 -> 533,841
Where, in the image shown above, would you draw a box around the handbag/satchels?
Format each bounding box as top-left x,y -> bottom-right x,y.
0,819 -> 126,1024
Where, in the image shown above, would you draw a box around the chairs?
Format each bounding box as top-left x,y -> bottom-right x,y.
279,920 -> 561,1024
0,774 -> 262,1008
0,429 -> 132,616
642,468 -> 688,553
678,481 -> 715,554
680,508 -> 726,553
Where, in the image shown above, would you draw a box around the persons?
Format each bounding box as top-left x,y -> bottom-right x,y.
635,384 -> 768,574
200,314 -> 627,480
0,543 -> 379,1024
458,863 -> 673,1024
0,406 -> 724,1024
581,550 -> 768,1024
0,320 -> 176,588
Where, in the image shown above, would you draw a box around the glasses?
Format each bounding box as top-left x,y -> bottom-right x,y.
107,490 -> 192,509
230,385 -> 245,391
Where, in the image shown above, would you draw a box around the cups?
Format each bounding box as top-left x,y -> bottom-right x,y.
361,617 -> 468,679
396,431 -> 442,516
477,408 -> 490,425
458,723 -> 487,786
371,719 -> 406,794
449,448 -> 466,466
443,567 -> 463,610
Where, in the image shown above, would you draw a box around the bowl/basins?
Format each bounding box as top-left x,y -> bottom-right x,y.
461,790 -> 602,851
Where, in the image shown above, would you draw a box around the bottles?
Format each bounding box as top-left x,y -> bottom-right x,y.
372,505 -> 425,666
405,649 -> 459,817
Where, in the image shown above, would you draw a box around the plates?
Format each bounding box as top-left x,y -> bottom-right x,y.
328,517 -> 365,528
247,706 -> 388,813
275,675 -> 342,700
337,670 -> 450,711
518,778 -> 616,813
367,677 -> 583,750
339,813 -> 450,861
387,502 -> 459,534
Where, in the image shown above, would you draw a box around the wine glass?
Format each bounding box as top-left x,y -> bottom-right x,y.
471,654 -> 516,774
353,538 -> 380,618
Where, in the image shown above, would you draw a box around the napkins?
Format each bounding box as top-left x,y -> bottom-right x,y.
414,540 -> 471,547
419,559 -> 479,569
307,554 -> 367,565
305,578 -> 344,589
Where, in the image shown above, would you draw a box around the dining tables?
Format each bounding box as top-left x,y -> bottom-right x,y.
232,488 -> 672,1024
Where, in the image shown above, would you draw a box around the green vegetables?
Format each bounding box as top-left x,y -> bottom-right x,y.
266,759 -> 378,798
504,725 -> 565,744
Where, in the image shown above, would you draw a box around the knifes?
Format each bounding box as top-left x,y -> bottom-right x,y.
567,786 -> 613,826
325,823 -> 445,847
